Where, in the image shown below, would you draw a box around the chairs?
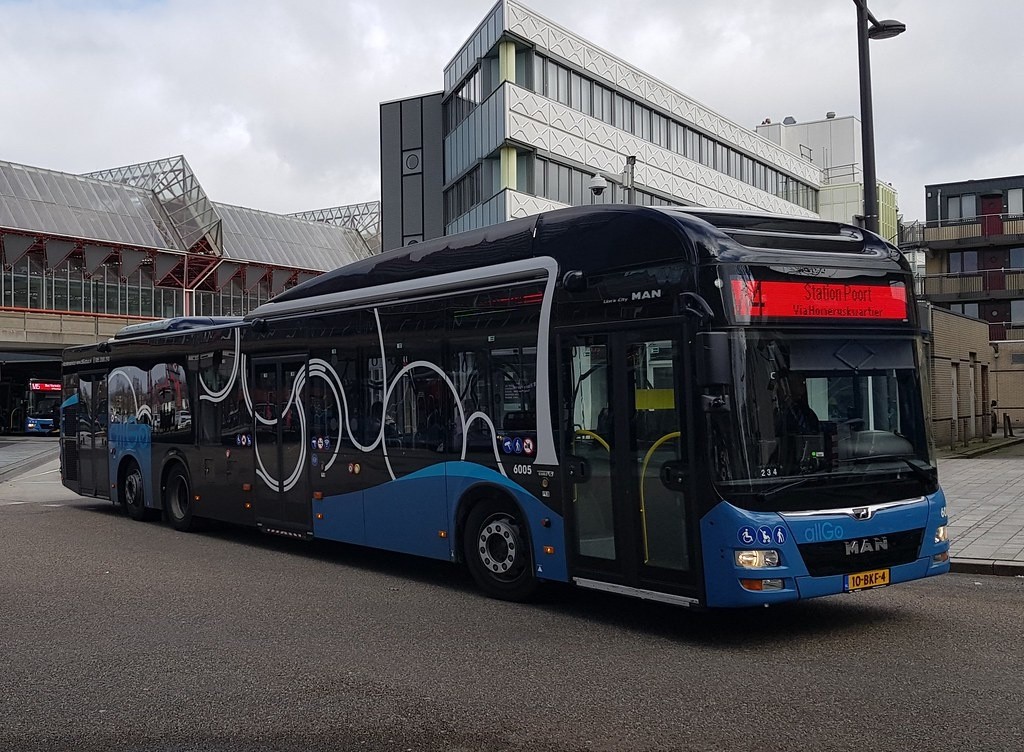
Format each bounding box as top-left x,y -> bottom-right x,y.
219,382 -> 696,459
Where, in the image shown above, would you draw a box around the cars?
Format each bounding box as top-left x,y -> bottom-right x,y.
79,420 -> 93,447
110,410 -> 125,423
173,409 -> 191,431
151,413 -> 170,433
93,420 -> 107,447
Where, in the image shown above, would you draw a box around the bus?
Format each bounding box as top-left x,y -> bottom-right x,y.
0,376 -> 61,436
56,200 -> 954,620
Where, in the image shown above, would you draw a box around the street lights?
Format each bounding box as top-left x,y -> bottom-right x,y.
853,0 -> 906,235
